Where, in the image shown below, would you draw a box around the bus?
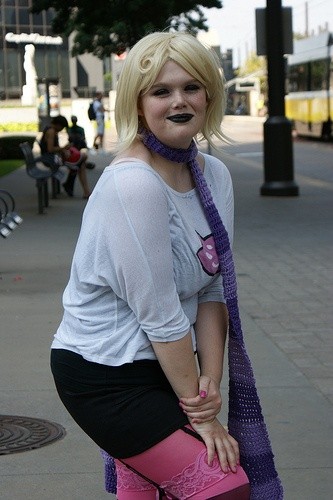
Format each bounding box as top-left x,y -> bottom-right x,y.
283,30 -> 333,142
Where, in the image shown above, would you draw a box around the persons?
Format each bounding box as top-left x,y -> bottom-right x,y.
50,31 -> 283,499
67,116 -> 85,146
93,92 -> 109,149
39,116 -> 96,196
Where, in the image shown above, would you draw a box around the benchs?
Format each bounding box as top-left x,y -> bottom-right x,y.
38,137 -> 76,199
19,142 -> 63,216
